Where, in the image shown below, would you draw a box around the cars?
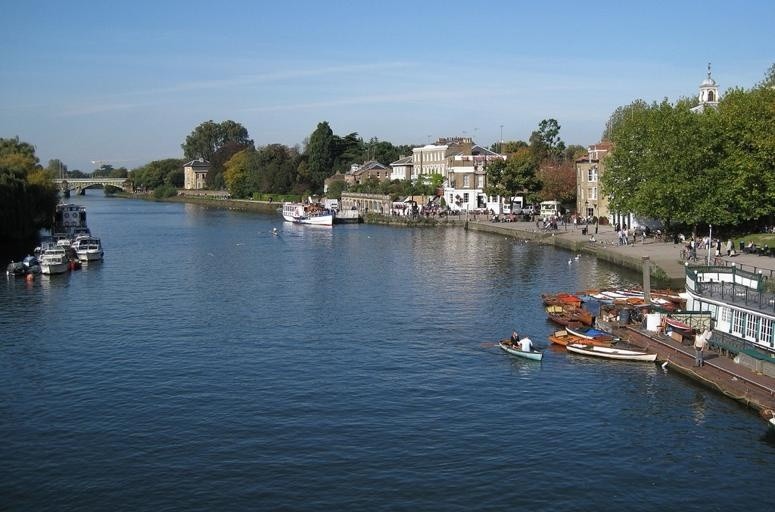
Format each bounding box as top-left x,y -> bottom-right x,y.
502,204 -> 539,215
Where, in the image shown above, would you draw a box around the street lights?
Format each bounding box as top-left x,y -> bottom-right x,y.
594,163 -> 599,228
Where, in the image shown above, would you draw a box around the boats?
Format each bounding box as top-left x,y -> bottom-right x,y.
499,339 -> 543,362
542,260 -> 775,429
6,203 -> 104,279
282,201 -> 334,226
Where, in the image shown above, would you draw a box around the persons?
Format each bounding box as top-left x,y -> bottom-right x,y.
511,329 -> 533,352
617,224 -> 663,245
672,232 -> 771,260
694,329 -> 706,367
391,201 -> 599,235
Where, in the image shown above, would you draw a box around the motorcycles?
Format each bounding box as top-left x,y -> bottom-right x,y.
679,248 -> 697,263
